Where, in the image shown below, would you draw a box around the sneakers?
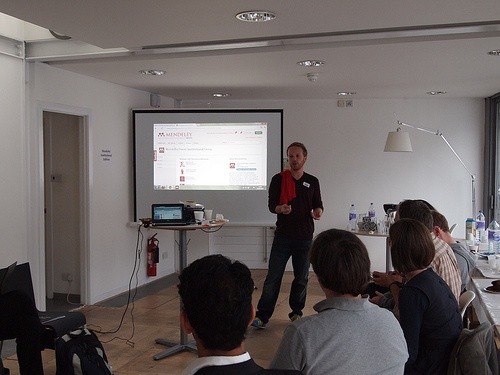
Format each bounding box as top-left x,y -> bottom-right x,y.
251,318 -> 266,329
290,314 -> 300,322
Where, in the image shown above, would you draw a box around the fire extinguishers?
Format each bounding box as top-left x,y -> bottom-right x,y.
147,233 -> 159,276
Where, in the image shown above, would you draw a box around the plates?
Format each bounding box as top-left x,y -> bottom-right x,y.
483,285 -> 500,293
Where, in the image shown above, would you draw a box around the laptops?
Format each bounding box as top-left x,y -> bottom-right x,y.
151,204 -> 187,225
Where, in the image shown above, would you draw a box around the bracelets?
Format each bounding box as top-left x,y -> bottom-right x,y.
388,281 -> 397,289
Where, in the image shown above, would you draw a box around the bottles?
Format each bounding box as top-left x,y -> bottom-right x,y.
383,212 -> 395,225
348,203 -> 357,232
368,202 -> 375,221
476,210 -> 486,254
488,217 -> 500,254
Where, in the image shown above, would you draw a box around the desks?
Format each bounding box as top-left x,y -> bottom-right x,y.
149,220 -> 224,360
453,238 -> 500,349
347,229 -> 395,278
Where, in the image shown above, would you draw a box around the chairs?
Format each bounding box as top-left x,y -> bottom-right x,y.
455,321 -> 491,375
459,290 -> 475,320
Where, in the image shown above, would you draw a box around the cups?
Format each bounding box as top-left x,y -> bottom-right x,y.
194,211 -> 204,223
488,254 -> 500,271
469,245 -> 479,255
216,214 -> 224,220
204,209 -> 212,220
370,217 -> 377,227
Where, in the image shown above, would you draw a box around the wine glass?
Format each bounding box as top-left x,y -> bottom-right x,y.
378,221 -> 390,235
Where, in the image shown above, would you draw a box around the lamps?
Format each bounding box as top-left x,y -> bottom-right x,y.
384,121 -> 476,218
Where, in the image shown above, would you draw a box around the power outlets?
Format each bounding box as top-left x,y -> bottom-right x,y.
163,252 -> 169,260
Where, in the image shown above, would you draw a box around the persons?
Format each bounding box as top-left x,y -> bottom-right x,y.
268,227 -> 409,375
177,253 -> 304,375
368,200 -> 462,324
250,141 -> 324,330
363,211 -> 477,296
387,217 -> 463,375
